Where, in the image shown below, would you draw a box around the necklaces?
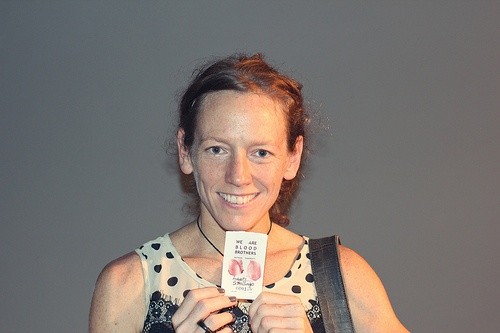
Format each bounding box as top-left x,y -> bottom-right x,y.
197,214 -> 272,258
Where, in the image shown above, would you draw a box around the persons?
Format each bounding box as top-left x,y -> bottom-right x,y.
88,52 -> 412,333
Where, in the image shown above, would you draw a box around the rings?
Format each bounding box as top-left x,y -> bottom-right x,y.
196,318 -> 216,333
